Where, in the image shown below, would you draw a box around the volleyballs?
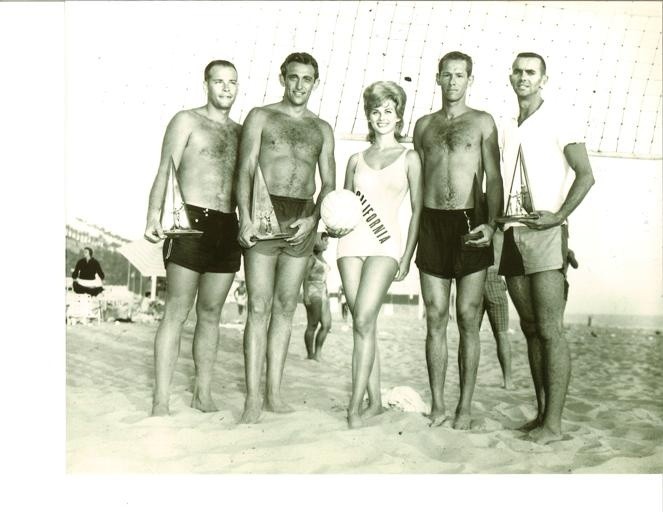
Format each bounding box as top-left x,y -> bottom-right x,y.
321,188 -> 363,229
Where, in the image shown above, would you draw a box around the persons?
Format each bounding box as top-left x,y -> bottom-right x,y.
480,221 -> 513,392
410,49 -> 503,435
301,231 -> 332,364
71,246 -> 105,298
142,58 -> 242,419
561,249 -> 578,318
499,52 -> 595,446
336,283 -> 348,322
322,80 -> 422,433
233,49 -> 337,429
138,291 -> 155,314
232,280 -> 247,315
